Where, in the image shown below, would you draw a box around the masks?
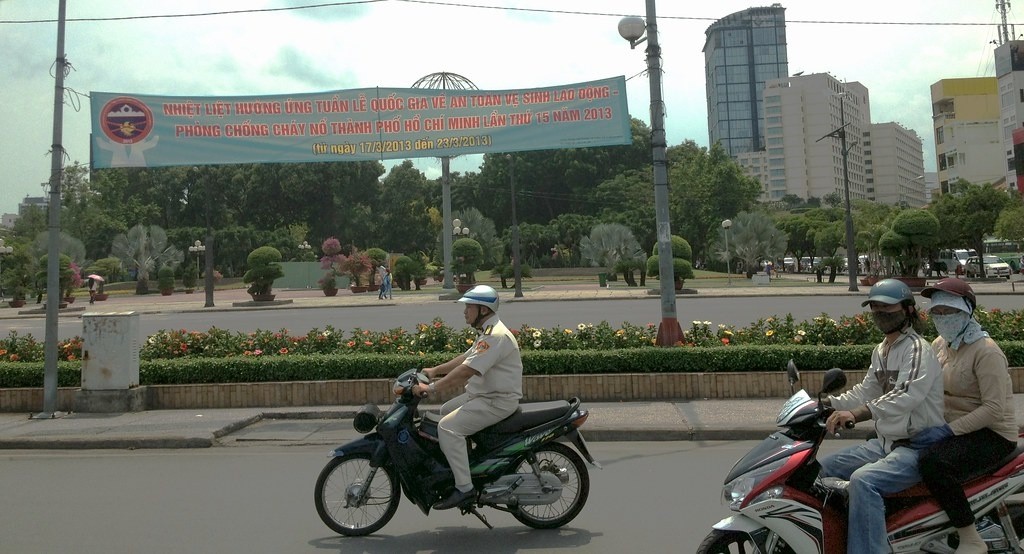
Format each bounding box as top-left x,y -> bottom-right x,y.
872,308 -> 910,335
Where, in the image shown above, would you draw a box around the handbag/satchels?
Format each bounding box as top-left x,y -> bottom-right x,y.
383,277 -> 388,286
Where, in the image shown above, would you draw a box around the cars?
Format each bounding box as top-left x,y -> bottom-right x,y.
785,255 -> 867,273
964,255 -> 1013,279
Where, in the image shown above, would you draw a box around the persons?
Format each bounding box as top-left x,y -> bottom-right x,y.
816,278 -> 947,554
857,259 -> 870,273
911,278 -> 1018,554
377,266 -> 392,300
88,277 -> 96,304
412,284 -> 524,510
936,262 -> 941,277
1020,254 -> 1024,274
956,260 -> 961,279
766,264 -> 771,276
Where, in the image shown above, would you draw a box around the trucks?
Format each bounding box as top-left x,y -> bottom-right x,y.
935,248 -> 977,275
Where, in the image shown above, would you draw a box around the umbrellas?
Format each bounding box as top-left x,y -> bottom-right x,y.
89,275 -> 104,281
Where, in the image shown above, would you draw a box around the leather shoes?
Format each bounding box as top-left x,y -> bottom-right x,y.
432,486 -> 476,510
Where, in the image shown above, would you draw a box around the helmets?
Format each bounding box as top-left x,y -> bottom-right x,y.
921,278 -> 977,307
861,278 -> 916,308
458,285 -> 499,314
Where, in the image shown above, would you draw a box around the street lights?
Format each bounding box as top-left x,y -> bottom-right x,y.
451,218 -> 469,243
298,241 -> 311,263
842,140 -> 859,291
617,16 -> 686,346
722,220 -> 731,283
506,154 -> 524,298
904,175 -> 925,209
189,240 -> 205,279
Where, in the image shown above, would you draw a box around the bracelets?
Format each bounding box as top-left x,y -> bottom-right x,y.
429,382 -> 435,393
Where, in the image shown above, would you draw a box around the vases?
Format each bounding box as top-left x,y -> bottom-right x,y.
351,287 -> 368,293
64,297 -> 75,303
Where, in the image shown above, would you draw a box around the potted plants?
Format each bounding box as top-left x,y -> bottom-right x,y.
449,238 -> 483,293
857,208 -> 941,287
363,248 -> 387,291
243,246 -> 284,301
322,289 -> 338,296
183,262 -> 200,294
158,267 -> 175,296
390,253 -> 427,288
647,236 -> 696,290
83,258 -> 128,301
0,268 -> 33,308
35,253 -> 75,309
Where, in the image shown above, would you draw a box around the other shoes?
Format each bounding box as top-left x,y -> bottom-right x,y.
948,523 -> 988,554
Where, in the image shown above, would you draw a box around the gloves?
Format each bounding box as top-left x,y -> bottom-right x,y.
909,423 -> 953,449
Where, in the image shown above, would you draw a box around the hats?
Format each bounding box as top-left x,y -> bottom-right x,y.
927,291 -> 972,316
386,268 -> 390,272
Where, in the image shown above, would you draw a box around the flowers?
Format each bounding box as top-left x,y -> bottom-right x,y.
317,237 -> 374,290
66,263 -> 84,297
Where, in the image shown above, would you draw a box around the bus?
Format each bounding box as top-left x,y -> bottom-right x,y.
982,239 -> 1024,271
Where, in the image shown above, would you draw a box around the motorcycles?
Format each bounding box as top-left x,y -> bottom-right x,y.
314,362 -> 603,537
696,359 -> 1024,554
